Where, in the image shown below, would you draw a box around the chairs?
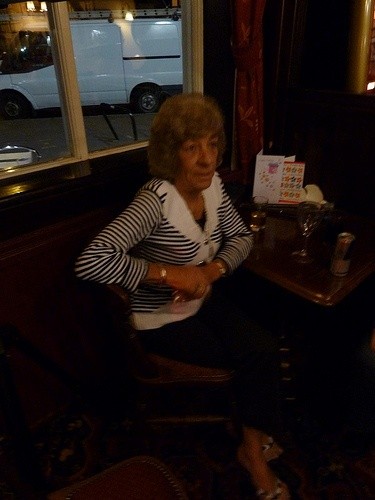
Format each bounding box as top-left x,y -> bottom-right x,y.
101,278 -> 240,460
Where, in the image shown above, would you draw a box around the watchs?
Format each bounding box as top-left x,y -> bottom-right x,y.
158,261 -> 166,286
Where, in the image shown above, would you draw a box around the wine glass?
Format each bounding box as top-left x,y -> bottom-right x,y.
290,201 -> 323,264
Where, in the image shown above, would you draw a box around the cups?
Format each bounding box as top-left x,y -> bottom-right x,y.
249,196 -> 269,232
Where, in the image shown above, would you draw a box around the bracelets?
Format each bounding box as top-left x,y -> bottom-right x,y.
212,259 -> 226,276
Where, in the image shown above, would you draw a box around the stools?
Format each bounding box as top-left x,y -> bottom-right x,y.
50,452 -> 189,499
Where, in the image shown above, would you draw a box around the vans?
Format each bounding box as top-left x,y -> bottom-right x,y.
0,16 -> 183,120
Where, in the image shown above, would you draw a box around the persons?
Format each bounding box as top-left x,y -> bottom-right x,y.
76,92 -> 295,500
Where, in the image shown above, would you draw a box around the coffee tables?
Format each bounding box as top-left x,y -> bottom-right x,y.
242,200 -> 375,311
